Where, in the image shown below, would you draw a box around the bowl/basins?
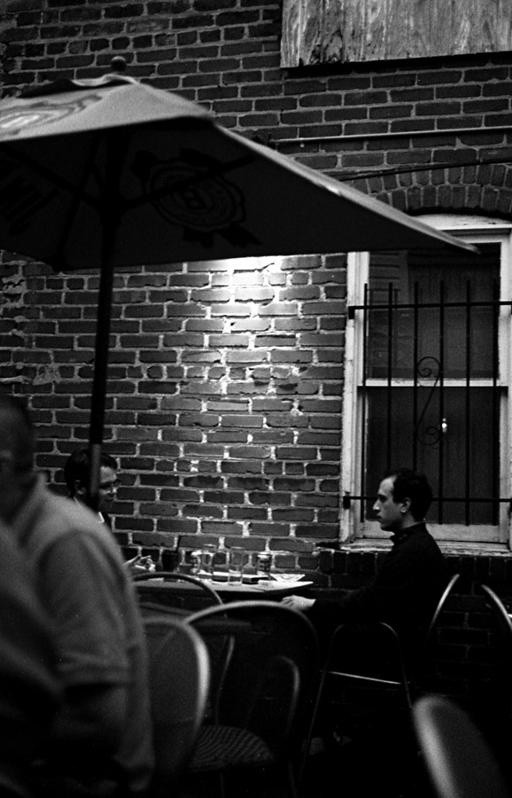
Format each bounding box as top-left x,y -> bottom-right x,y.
179,564 -> 199,575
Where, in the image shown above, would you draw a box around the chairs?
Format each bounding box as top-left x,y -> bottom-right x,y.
152,598 -> 326,797
411,693 -> 511,798
131,569 -> 236,726
300,569 -> 463,773
480,583 -> 512,633
139,614 -> 211,795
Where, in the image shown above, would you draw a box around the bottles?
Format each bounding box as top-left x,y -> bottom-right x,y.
210,534 -> 230,579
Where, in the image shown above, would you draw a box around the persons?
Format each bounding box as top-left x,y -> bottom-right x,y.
66,446 -> 154,575
0,392 -> 156,797
280,469 -> 444,675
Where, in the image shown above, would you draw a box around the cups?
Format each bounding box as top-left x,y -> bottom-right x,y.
161,551 -> 181,582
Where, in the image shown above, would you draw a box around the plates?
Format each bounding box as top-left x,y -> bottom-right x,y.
273,573 -> 305,582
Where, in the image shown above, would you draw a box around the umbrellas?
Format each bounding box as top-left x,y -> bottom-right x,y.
0,56 -> 479,511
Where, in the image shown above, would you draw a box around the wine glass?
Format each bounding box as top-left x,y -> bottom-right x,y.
245,551 -> 257,575
